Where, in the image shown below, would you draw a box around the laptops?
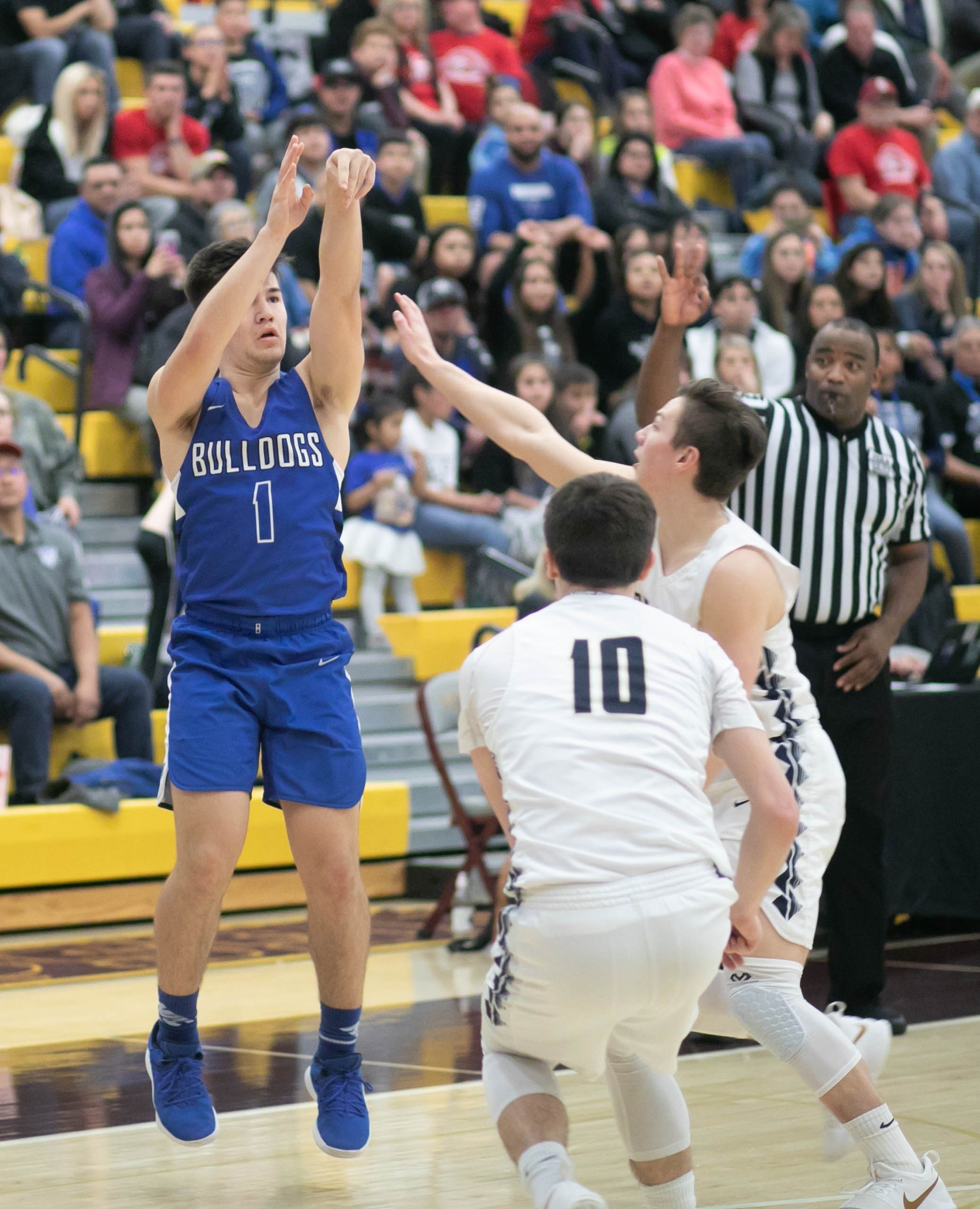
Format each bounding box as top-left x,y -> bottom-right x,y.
917,618 -> 979,685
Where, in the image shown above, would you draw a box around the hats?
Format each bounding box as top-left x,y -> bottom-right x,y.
190,148 -> 238,178
860,76 -> 898,102
415,276 -> 469,315
321,57 -> 362,82
0,439 -> 22,454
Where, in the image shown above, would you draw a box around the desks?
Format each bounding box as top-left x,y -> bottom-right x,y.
868,684 -> 980,924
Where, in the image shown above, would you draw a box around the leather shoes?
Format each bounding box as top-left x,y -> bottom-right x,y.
866,1004 -> 907,1035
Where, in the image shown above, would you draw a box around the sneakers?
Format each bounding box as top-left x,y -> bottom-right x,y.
145,1019 -> 219,1147
839,1150 -> 958,1209
819,1000 -> 892,1162
305,1050 -> 369,1157
538,1179 -> 607,1209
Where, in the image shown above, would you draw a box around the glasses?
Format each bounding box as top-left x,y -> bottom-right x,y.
323,74 -> 361,87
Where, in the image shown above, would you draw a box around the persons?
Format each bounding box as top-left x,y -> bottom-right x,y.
145,134 -> 379,1157
635,241 -> 933,1032
391,292 -> 955,1209
458,472 -> 800,1209
0,0 -> 980,808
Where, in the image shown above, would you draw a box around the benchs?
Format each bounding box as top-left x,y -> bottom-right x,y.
0,0 -> 980,892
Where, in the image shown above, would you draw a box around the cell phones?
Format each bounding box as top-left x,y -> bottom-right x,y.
160,228 -> 181,253
